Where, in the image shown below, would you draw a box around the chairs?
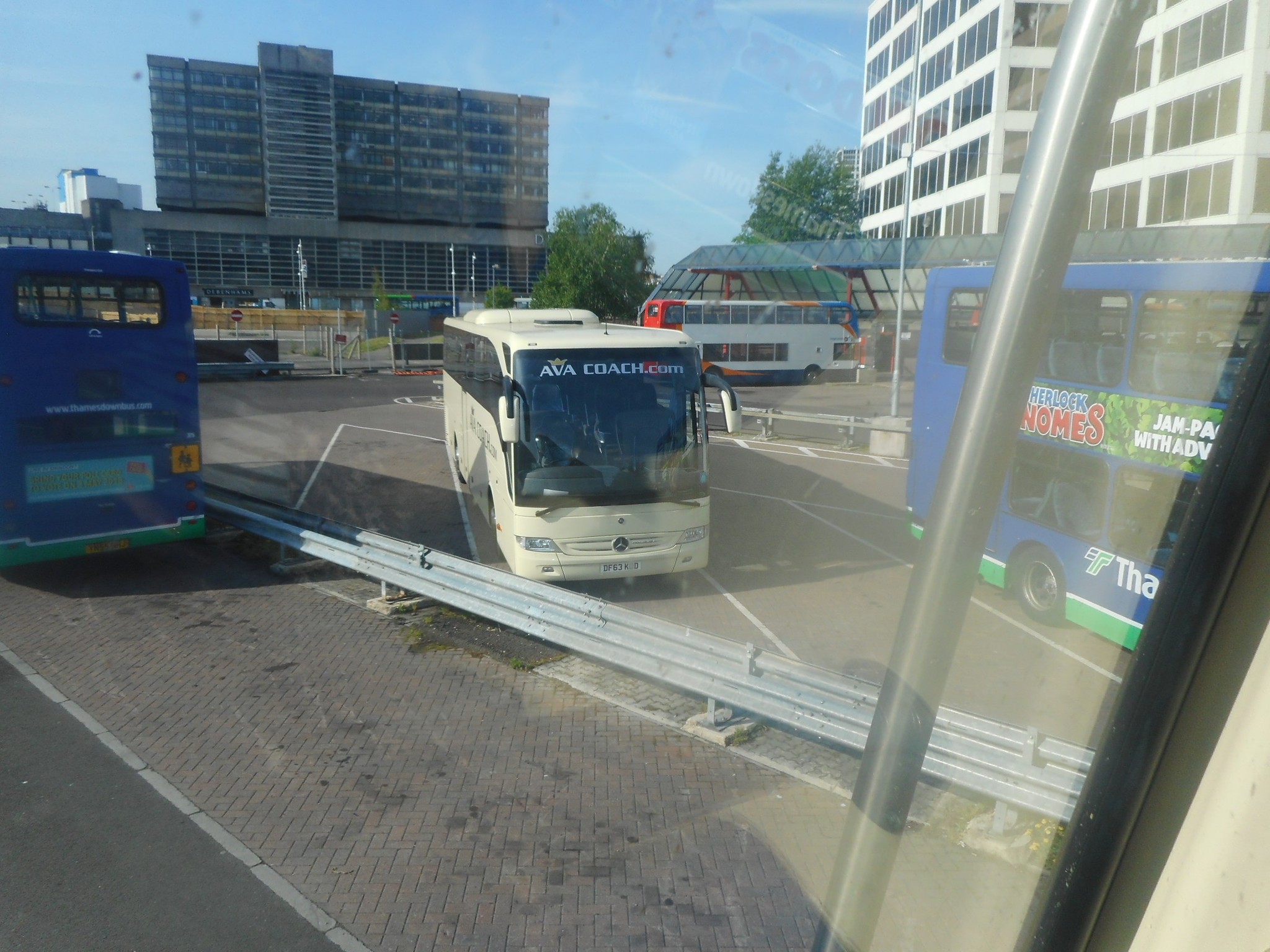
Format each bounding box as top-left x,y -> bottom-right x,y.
1013,330 -> 1254,567
531,376 -> 657,469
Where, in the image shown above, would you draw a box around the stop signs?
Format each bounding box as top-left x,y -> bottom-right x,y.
230,309 -> 243,321
390,313 -> 400,323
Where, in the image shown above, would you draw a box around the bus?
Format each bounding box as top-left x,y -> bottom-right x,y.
441,303 -> 714,593
645,298 -> 862,386
0,243 -> 212,570
909,263 -> 1270,652
